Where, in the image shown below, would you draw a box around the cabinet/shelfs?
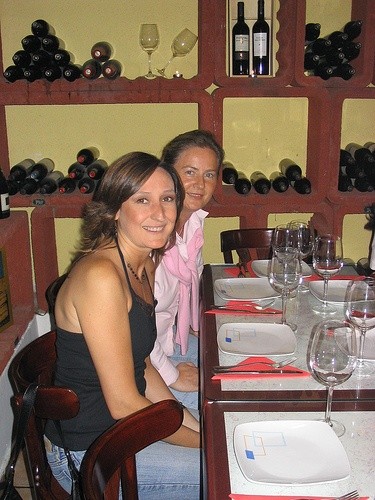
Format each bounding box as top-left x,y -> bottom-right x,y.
0,0 -> 375,374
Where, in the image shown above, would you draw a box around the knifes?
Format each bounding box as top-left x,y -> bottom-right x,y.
211,369 -> 303,375
211,306 -> 284,314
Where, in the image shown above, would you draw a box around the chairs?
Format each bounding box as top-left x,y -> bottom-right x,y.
220,228 -> 323,264
7,328 -> 184,500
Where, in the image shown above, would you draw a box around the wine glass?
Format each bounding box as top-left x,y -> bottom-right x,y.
272,224 -> 303,300
269,247 -> 302,332
308,319 -> 358,438
343,275 -> 375,378
155,27 -> 198,79
312,234 -> 343,315
138,23 -> 160,78
287,220 -> 315,292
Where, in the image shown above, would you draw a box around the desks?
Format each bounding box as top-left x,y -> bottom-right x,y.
198,258 -> 375,500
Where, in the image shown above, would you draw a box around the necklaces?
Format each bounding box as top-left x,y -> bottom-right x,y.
125,259 -> 146,284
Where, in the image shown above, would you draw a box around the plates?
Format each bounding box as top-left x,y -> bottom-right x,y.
309,279 -> 375,306
232,420 -> 351,487
214,277 -> 282,302
216,322 -> 297,357
252,259 -> 313,279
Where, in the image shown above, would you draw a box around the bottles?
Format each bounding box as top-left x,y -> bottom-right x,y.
3,18 -> 123,82
0,146 -> 108,219
232,2 -> 250,76
252,0 -> 270,75
222,158 -> 312,195
338,142 -> 375,192
304,19 -> 362,81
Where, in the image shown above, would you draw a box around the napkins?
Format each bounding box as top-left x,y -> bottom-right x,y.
211,357 -> 311,379
303,273 -> 366,282
324,310 -> 375,336
224,267 -> 245,279
205,301 -> 283,316
228,493 -> 370,500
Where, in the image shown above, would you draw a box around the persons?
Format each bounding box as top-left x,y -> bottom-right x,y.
42,152 -> 201,500
149,128 -> 227,410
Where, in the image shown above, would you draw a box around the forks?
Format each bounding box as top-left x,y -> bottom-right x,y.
214,356 -> 298,371
297,490 -> 360,500
211,298 -> 276,310
